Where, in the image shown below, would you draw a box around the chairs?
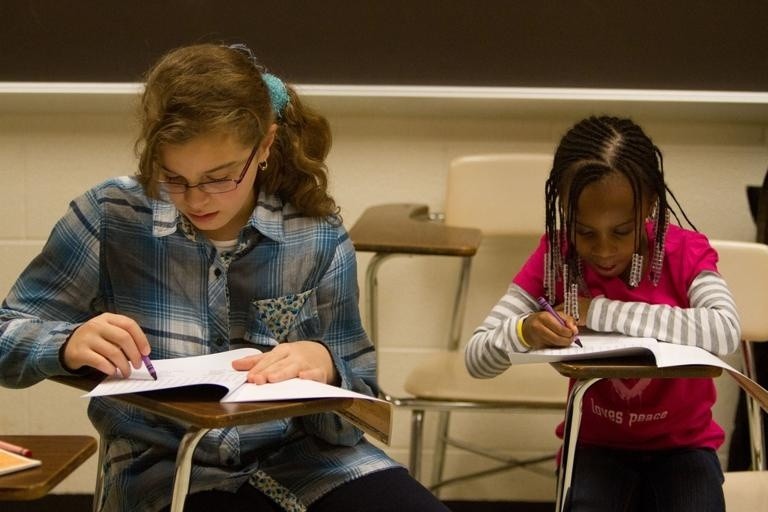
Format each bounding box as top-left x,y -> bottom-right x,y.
689,240 -> 768,512
405,150 -> 573,511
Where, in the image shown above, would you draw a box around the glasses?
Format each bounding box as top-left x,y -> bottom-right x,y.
154,138 -> 262,194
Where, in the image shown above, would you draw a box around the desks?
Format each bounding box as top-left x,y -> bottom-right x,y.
1,431 -> 98,506
102,387 -> 357,512
554,337 -> 723,512
348,199 -> 484,412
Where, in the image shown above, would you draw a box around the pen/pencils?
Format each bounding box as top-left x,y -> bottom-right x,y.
141,353 -> 157,381
0,440 -> 33,457
536,296 -> 583,348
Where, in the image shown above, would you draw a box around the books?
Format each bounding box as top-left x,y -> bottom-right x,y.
0,447 -> 41,475
80,346 -> 394,448
507,332 -> 768,415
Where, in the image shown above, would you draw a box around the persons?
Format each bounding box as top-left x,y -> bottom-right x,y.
463,113 -> 742,512
0,41 -> 454,511
